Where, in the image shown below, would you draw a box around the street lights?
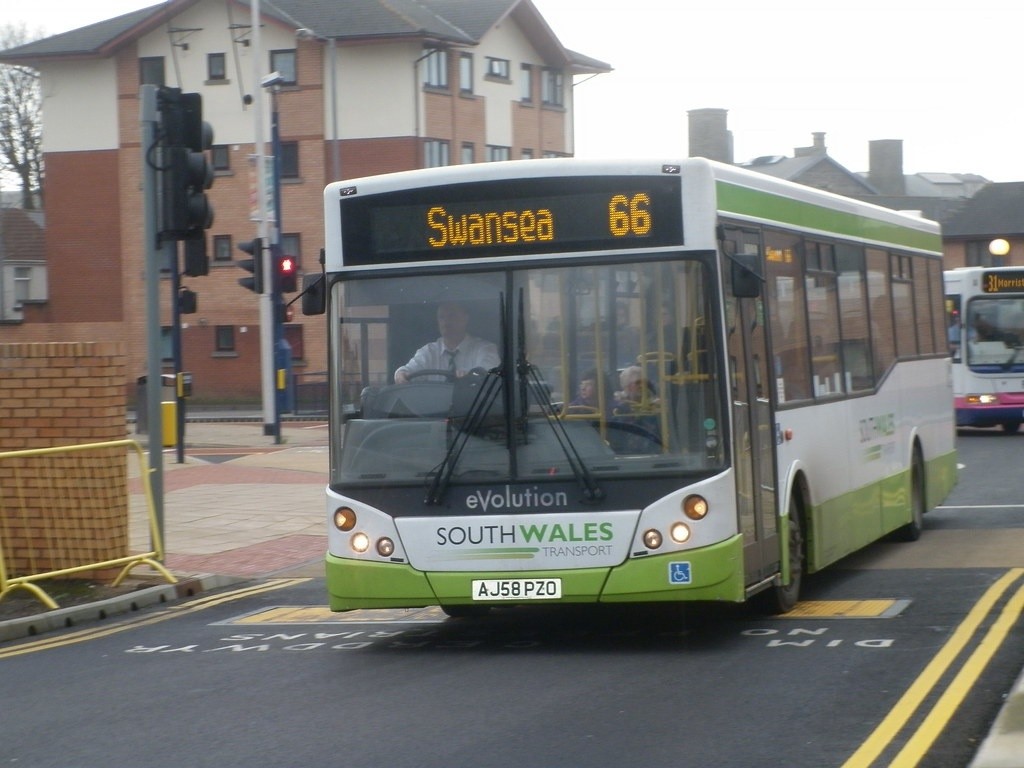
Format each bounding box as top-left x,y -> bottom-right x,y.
291,26 -> 341,182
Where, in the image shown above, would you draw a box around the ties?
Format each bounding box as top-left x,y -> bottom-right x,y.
446,349 -> 458,371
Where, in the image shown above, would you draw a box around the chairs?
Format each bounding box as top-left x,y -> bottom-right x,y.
638,351 -> 674,399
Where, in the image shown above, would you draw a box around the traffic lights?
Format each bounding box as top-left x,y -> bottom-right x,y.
277,254 -> 297,293
236,238 -> 263,294
185,123 -> 215,235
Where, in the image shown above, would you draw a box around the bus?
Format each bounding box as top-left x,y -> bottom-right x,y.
301,154 -> 961,622
769,266 -> 1024,435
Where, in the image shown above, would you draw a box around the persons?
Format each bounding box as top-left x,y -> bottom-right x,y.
543,299 -> 698,416
393,301 -> 501,382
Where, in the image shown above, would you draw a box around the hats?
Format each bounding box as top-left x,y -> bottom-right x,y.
619,365 -> 641,387
